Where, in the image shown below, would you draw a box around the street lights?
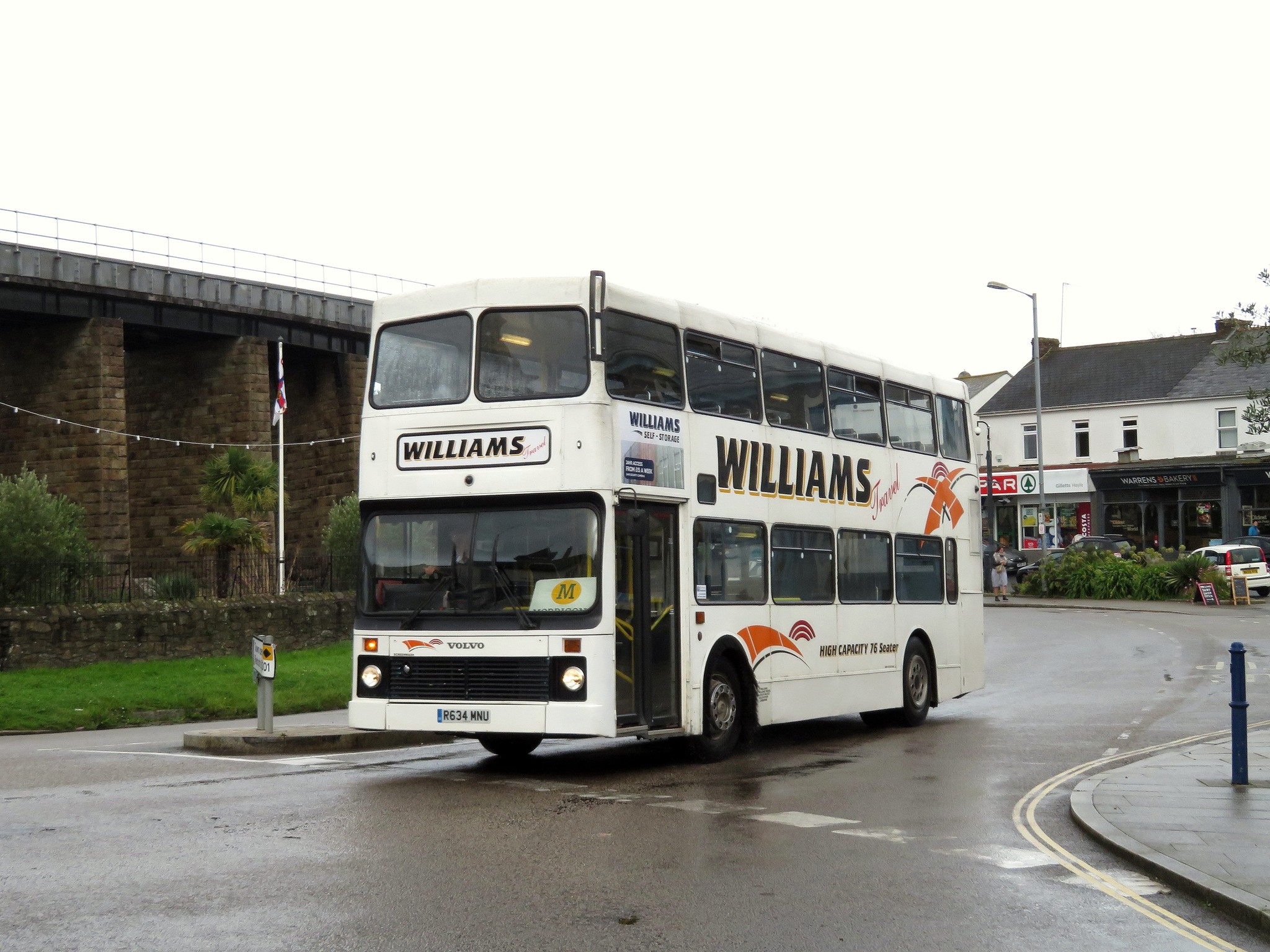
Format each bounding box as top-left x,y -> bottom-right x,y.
986,280 -> 1046,592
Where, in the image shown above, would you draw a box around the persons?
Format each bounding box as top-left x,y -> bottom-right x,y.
422,552 -> 468,608
1248,519 -> 1260,537
989,545 -> 1009,602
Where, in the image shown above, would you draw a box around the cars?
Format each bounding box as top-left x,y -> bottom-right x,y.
1016,552 -> 1068,583
1186,545 -> 1270,596
981,538 -> 1028,572
1223,537 -> 1270,564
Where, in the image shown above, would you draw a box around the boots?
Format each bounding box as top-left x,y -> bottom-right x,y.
995,596 -> 1000,602
1002,594 -> 1008,601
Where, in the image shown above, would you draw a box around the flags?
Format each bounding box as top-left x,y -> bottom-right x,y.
271,349 -> 289,427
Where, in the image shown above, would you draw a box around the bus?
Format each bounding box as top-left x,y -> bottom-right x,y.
345,270 -> 988,765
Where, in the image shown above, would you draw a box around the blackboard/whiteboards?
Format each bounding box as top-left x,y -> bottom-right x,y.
1193,583 -> 1220,606
1231,577 -> 1250,599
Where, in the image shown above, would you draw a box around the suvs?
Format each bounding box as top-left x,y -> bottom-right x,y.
1061,536 -> 1141,563
